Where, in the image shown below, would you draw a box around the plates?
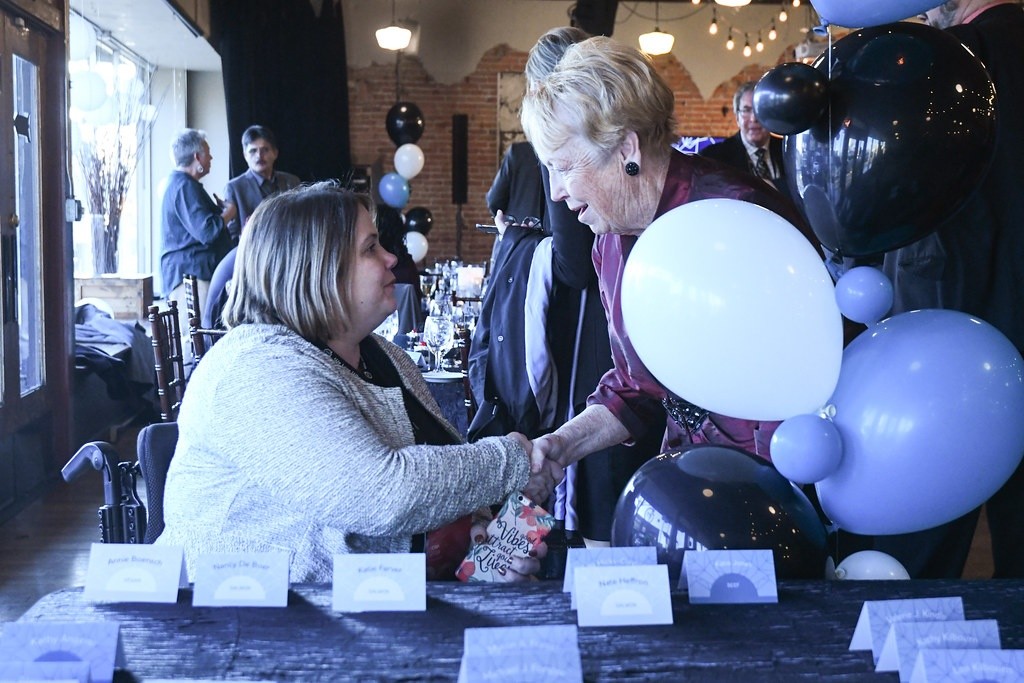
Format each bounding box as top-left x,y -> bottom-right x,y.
418,371 -> 465,384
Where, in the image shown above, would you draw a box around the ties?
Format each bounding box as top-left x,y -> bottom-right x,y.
756,149 -> 773,182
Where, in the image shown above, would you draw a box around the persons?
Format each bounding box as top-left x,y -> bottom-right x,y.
160,129 -> 237,394
376,203 -> 427,300
836,0 -> 1024,576
486,141 -> 550,225
694,79 -> 787,186
495,26 -> 666,540
153,178 -> 564,586
224,126 -> 302,245
532,27 -> 984,578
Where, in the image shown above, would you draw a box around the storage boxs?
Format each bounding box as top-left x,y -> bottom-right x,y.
74,275 -> 153,319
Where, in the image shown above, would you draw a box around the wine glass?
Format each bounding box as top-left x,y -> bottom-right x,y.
420,255 -> 487,377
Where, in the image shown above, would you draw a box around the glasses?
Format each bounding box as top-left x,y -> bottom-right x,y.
504,214 -> 542,228
737,107 -> 756,117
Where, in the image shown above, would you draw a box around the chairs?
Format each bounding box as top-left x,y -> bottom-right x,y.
189,318 -> 228,366
147,299 -> 186,422
183,273 -> 212,357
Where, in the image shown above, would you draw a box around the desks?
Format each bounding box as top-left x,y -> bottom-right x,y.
0,582 -> 1024,683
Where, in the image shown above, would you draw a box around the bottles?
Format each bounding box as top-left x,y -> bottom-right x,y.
433,262 -> 444,289
463,305 -> 476,330
453,300 -> 465,333
449,260 -> 458,294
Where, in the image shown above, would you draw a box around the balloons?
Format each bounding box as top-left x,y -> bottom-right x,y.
379,173 -> 410,207
753,23 -> 999,257
69,14 -> 159,127
394,144 -> 425,180
620,196 -> 894,484
404,232 -> 428,261
807,0 -> 953,36
405,207 -> 434,234
818,308 -> 1024,534
612,445 -> 828,577
385,102 -> 425,147
835,551 -> 912,579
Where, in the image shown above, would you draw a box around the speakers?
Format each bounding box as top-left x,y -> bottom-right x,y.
576,0 -> 618,37
451,114 -> 468,205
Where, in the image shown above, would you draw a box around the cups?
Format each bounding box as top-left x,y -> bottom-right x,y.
370,309 -> 399,344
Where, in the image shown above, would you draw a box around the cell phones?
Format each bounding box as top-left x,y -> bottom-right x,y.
456,492 -> 556,584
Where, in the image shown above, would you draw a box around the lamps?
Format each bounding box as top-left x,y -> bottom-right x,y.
376,0 -> 411,50
639,1 -> 676,56
692,0 -> 800,57
